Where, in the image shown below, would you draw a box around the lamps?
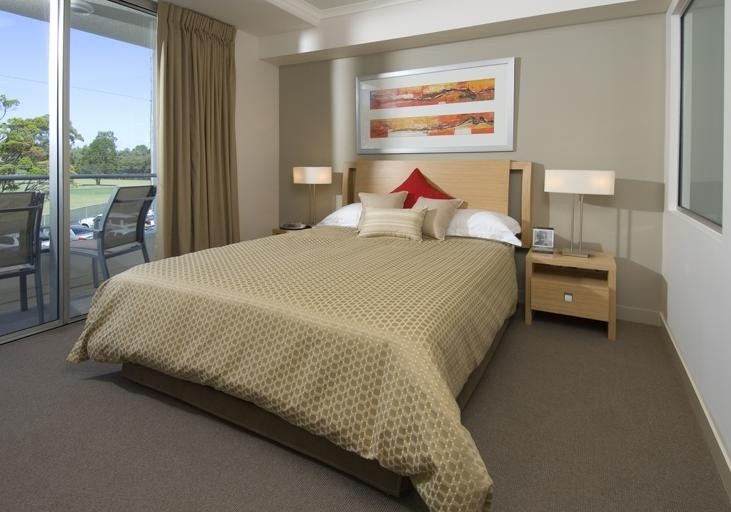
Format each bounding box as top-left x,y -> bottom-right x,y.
544,169 -> 615,258
293,166 -> 332,226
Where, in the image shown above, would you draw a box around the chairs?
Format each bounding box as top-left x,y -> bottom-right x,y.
0,192 -> 45,324
69,186 -> 156,289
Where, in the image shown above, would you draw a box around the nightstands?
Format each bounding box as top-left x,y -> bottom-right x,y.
273,225 -> 311,235
525,247 -> 617,341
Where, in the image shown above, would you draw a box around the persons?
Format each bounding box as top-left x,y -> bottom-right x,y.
535,231 -> 548,246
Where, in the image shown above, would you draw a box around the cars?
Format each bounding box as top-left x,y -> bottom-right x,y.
39,209 -> 155,255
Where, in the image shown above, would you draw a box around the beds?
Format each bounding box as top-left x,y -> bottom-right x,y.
66,159 -> 533,512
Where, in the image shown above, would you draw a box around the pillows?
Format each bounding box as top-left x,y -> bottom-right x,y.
311,168 -> 522,247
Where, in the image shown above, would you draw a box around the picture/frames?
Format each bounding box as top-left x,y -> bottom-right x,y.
356,57 -> 515,154
